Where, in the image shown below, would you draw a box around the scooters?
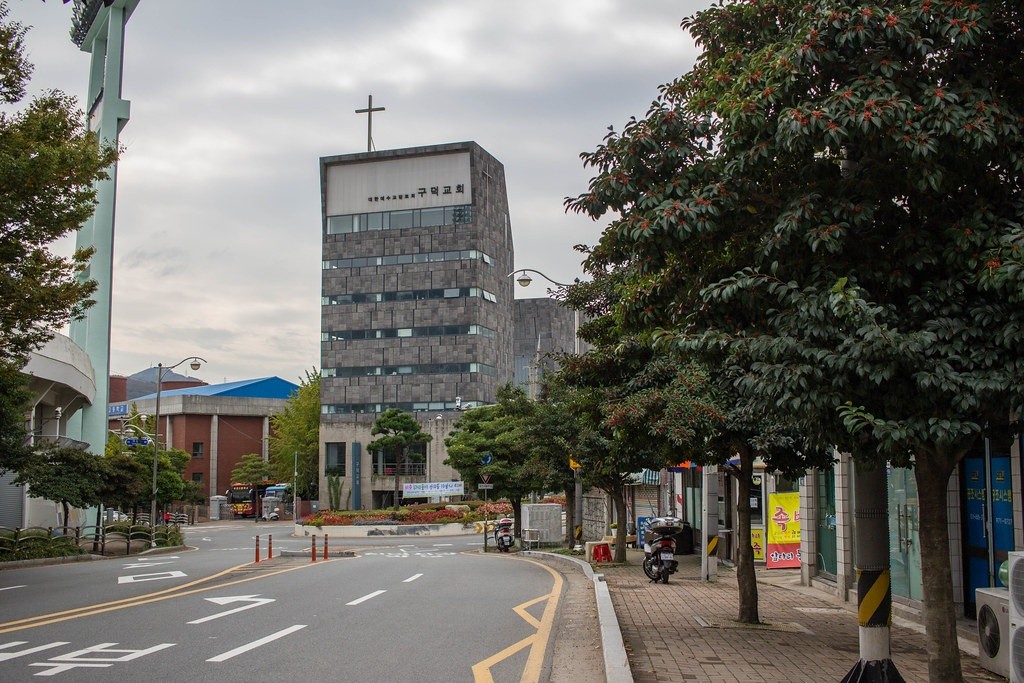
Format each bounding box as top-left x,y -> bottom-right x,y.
262,508 -> 280,522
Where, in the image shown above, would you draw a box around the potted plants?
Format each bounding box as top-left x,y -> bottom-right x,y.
610,523 -> 617,536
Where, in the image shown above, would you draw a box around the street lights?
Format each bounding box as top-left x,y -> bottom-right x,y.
507,269 -> 582,557
149,357 -> 207,548
116,412 -> 151,532
261,437 -> 299,521
427,414 -> 443,504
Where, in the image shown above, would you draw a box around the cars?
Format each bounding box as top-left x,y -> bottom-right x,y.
102,510 -> 150,526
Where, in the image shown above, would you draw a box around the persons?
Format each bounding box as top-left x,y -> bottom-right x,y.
163,510 -> 170,525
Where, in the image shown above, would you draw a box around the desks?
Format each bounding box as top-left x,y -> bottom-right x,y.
585,541 -> 612,563
603,535 -> 636,548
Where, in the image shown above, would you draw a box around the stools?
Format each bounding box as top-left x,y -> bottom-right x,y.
592,544 -> 612,562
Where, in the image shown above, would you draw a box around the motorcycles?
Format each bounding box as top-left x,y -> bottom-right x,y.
643,517 -> 684,584
487,518 -> 514,552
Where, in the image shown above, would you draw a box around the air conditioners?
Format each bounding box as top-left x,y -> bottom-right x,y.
1007,550 -> 1024,683
976,587 -> 1011,678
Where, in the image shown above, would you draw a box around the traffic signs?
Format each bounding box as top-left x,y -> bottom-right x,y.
478,484 -> 492,489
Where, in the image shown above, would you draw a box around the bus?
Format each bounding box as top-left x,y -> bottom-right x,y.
224,480 -> 284,518
260,483 -> 319,520
